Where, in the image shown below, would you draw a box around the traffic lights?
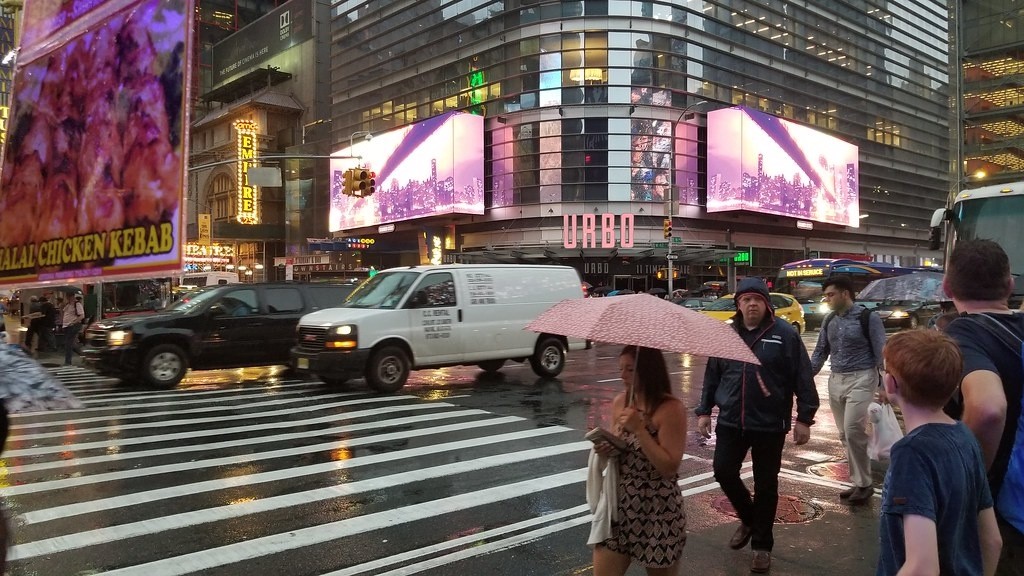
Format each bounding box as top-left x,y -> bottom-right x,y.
657,270 -> 665,280
664,219 -> 672,239
673,269 -> 679,279
342,171 -> 353,196
352,168 -> 376,197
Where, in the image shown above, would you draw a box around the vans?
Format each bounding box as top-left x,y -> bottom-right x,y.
288,265 -> 593,393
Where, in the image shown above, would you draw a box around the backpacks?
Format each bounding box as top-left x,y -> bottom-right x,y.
953,312 -> 1024,533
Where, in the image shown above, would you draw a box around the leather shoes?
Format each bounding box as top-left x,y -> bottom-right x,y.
729,522 -> 753,550
751,549 -> 770,573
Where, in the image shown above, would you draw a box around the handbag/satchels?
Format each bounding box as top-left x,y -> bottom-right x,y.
866,402 -> 904,462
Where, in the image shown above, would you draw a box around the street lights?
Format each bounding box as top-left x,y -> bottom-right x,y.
944,172 -> 986,250
667,100 -> 708,300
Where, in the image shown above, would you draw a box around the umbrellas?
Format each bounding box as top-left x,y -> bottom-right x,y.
673,289 -> 688,293
583,281 -> 666,296
525,294 -> 761,409
44,286 -> 84,298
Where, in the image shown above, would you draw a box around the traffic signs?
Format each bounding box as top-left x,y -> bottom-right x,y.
665,254 -> 680,261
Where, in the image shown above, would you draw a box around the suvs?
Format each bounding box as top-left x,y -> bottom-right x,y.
82,281 -> 362,390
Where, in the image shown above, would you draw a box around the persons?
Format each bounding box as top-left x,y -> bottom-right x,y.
809,276 -> 887,503
695,276 -> 820,574
592,346 -> 688,576
943,239 -> 1024,576
876,328 -> 1003,576
25,292 -> 85,368
664,292 -> 682,299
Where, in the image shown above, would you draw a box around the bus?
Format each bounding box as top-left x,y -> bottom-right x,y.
772,257 -> 940,330
928,180 -> 1024,309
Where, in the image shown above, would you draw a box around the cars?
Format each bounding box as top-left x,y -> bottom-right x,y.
869,298 -> 941,331
677,291 -> 806,336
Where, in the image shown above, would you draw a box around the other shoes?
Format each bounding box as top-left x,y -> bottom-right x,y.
840,486 -> 857,498
849,484 -> 875,501
63,363 -> 72,368
